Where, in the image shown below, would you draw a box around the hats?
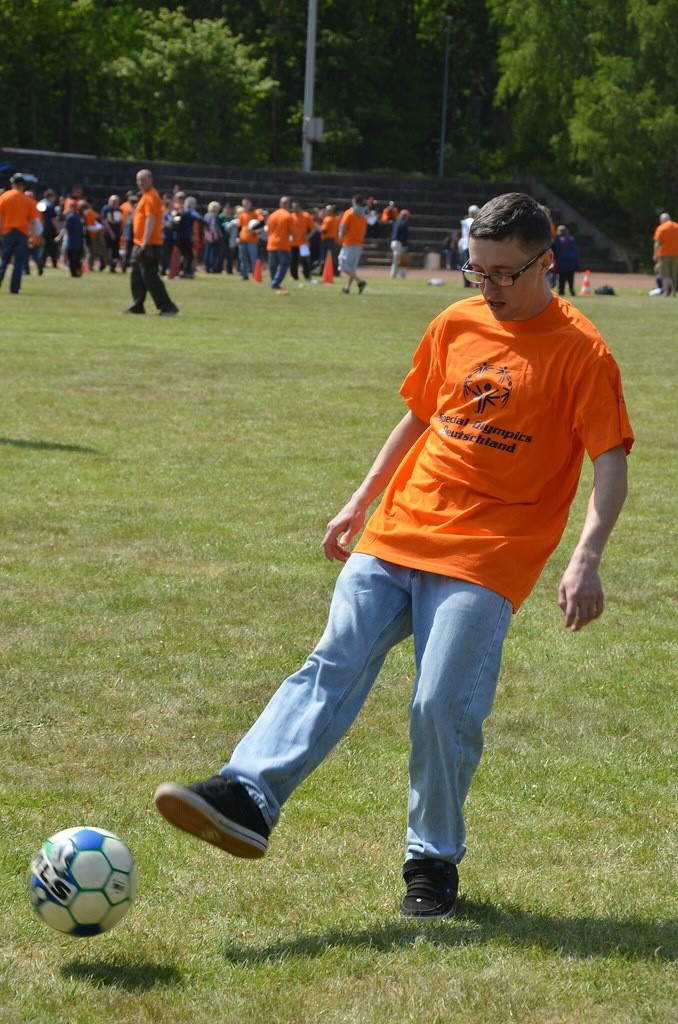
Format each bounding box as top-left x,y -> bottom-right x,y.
468,206 -> 481,216
9,173 -> 25,184
400,209 -> 409,217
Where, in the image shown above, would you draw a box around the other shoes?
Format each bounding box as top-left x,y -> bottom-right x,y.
159,309 -> 178,317
340,288 -> 349,294
123,306 -> 145,314
358,281 -> 367,295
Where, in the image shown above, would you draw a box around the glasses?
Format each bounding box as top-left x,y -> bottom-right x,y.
461,248 -> 548,288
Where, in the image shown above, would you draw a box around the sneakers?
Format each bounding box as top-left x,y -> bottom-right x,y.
397,859 -> 460,921
155,776 -> 270,859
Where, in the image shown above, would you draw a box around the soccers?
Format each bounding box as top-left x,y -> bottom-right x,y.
27,824 -> 143,940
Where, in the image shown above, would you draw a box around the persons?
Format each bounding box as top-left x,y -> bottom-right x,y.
151,195 -> 635,922
1,162 -> 677,328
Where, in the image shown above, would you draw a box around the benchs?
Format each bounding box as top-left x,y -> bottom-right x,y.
172,175 -> 628,272
